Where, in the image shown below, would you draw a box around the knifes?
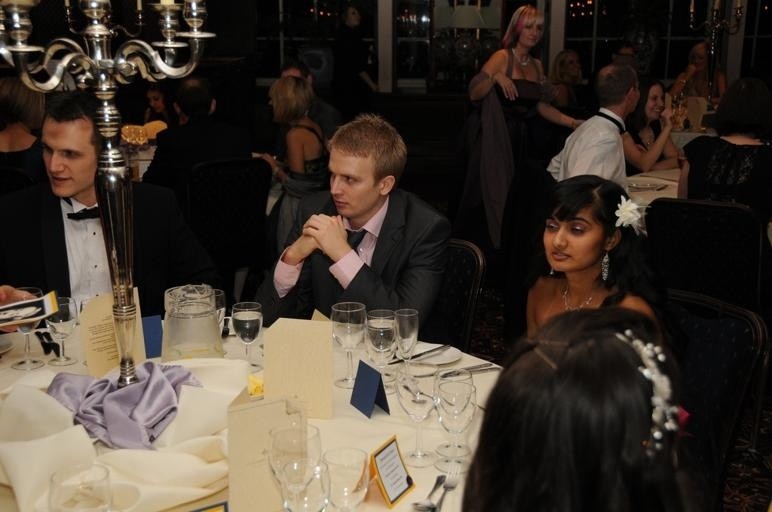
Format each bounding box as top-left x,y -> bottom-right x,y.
388,343 -> 450,365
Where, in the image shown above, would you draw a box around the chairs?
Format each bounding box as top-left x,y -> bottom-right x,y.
417,237 -> 485,353
630,281 -> 769,511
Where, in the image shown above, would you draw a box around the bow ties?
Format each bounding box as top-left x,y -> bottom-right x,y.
62,197 -> 100,219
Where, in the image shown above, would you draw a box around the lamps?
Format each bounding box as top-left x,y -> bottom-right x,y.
0,1 -> 216,389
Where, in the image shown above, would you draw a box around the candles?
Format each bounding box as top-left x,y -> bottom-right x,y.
734,1 -> 741,15
713,1 -> 720,20
690,0 -> 695,28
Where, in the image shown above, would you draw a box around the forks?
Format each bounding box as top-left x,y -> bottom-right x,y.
435,464 -> 460,510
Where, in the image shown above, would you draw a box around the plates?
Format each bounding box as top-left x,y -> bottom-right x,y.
472,377 -> 494,409
394,341 -> 462,365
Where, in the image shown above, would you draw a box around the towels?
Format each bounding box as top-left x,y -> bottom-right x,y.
1,359 -> 253,511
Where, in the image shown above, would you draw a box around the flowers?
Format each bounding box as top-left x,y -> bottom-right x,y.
615,193 -> 651,237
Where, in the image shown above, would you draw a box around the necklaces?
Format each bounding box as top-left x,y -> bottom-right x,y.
511,46 -> 531,71
559,280 -> 603,314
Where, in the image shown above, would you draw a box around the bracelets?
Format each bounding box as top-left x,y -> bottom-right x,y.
678,79 -> 688,84
488,73 -> 495,84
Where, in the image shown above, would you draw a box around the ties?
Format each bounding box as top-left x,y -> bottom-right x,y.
345,229 -> 368,256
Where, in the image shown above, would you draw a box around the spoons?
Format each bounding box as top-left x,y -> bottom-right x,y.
412,474 -> 445,510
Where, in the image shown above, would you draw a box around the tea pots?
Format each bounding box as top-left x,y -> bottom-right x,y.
162,284 -> 224,362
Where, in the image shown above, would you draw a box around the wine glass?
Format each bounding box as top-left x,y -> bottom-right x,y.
330,302 -> 368,390
231,302 -> 263,360
433,367 -> 474,457
10,288 -> 78,371
432,382 -> 477,475
367,323 -> 398,393
394,361 -> 439,469
368,309 -> 396,382
677,150 -> 685,170
672,94 -> 687,127
394,308 -> 418,374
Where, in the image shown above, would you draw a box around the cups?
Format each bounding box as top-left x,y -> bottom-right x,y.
321,447 -> 370,510
281,458 -> 330,511
49,463 -> 111,512
270,422 -> 322,496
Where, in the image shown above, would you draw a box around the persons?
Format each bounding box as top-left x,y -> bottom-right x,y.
250,114 -> 459,344
675,76 -> 772,317
469,6 -> 585,332
460,309 -> 687,510
3,0 -> 383,333
541,36 -> 729,200
526,173 -> 665,384
